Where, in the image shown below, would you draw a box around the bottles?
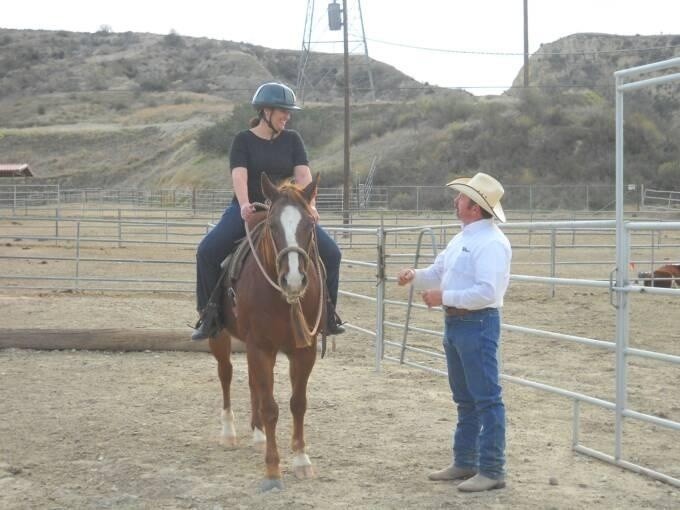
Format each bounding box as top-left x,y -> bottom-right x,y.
446,171 -> 510,223
251,83 -> 302,111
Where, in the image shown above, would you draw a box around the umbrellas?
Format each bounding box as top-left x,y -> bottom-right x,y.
428,464 -> 476,480
457,473 -> 506,493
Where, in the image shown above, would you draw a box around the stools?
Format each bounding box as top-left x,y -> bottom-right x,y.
446,306 -> 492,317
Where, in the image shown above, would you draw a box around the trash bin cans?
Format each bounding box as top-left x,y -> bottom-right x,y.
189,81 -> 346,342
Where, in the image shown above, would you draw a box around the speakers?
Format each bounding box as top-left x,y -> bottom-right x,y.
326,298 -> 347,335
191,308 -> 222,340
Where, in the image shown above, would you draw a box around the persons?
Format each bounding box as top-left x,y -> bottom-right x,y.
396,169 -> 514,493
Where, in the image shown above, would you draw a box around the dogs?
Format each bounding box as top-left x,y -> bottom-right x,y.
635,263 -> 680,289
208,169 -> 331,495
